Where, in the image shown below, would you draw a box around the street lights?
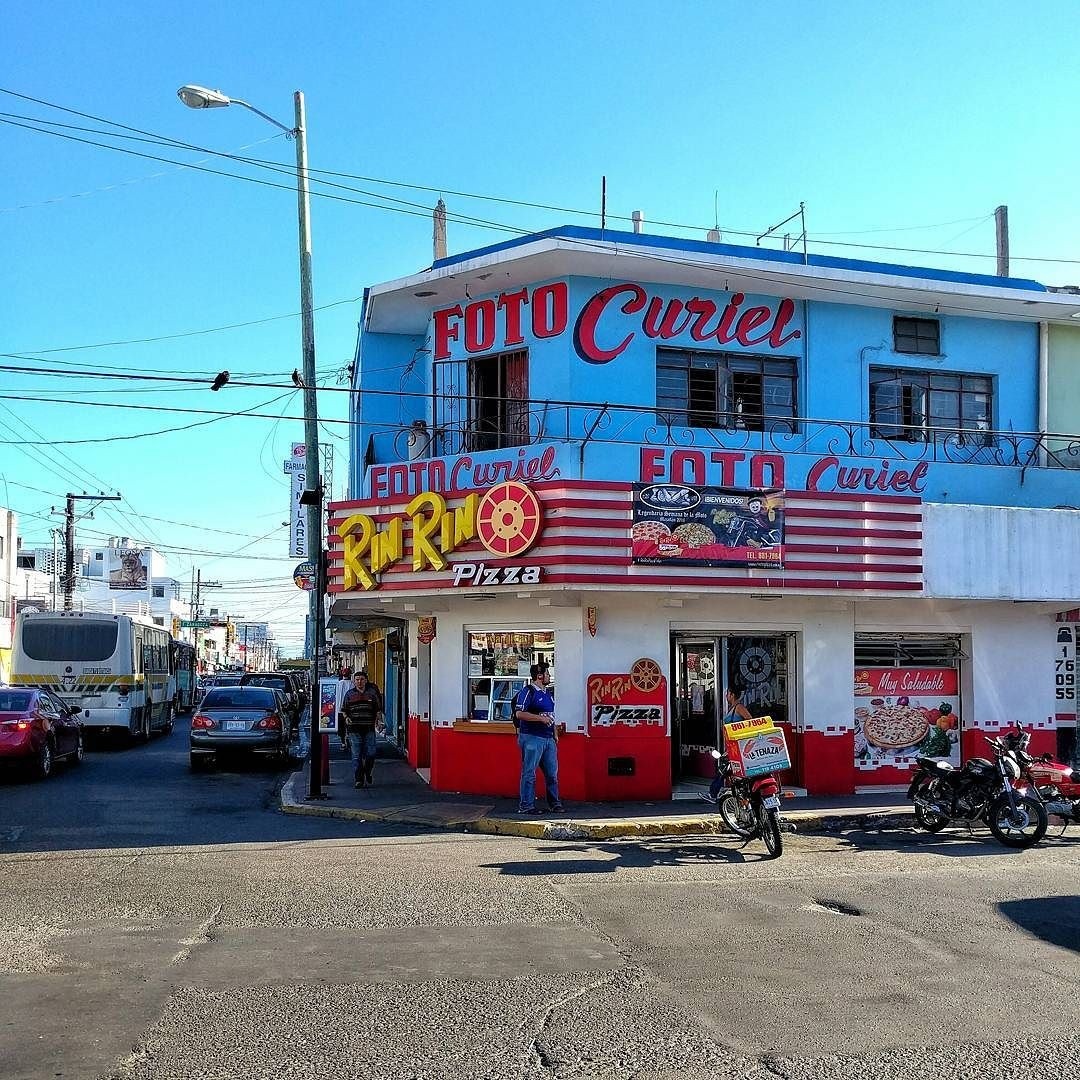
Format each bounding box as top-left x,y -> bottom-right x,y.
177,83 -> 330,803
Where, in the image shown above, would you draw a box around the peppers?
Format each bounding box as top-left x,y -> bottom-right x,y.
917,702 -> 958,730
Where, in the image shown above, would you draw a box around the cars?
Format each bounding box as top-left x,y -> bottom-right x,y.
0,686 -> 86,781
196,669 -> 310,742
190,685 -> 296,773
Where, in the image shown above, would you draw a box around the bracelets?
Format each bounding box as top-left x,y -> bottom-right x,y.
344,715 -> 349,721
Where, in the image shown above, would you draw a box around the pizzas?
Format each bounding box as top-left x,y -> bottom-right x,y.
863,705 -> 930,749
633,520 -> 671,541
671,523 -> 716,545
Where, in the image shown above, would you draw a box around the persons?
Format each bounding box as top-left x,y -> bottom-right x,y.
511,663 -> 565,815
698,685 -> 752,804
330,664 -> 385,789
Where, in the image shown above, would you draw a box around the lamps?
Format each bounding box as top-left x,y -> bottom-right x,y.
465,594 -> 498,603
750,594 -> 785,602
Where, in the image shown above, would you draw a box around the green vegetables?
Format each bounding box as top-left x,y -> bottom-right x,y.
921,726 -> 951,758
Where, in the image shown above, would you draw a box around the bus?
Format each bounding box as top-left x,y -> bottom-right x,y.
9,612 -> 180,748
173,640 -> 198,714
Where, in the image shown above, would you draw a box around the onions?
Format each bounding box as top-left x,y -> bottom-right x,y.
897,697 -> 909,705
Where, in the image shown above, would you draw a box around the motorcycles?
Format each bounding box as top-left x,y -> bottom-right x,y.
906,731 -> 1049,849
710,746 -> 798,858
978,719 -> 1080,838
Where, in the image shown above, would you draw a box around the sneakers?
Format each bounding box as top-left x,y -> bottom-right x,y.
698,792 -> 717,804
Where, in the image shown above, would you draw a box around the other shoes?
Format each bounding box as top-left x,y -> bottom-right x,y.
355,782 -> 364,789
365,772 -> 372,783
340,744 -> 345,750
551,805 -> 566,814
519,807 -> 548,816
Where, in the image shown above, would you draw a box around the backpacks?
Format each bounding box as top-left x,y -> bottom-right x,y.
510,685 -> 535,728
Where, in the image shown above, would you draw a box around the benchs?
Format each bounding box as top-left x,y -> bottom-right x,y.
217,696 -> 270,707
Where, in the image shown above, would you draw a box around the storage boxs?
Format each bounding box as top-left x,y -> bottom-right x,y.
723,715 -> 792,778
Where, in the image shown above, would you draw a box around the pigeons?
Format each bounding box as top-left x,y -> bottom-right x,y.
211,370 -> 231,391
292,369 -> 306,388
